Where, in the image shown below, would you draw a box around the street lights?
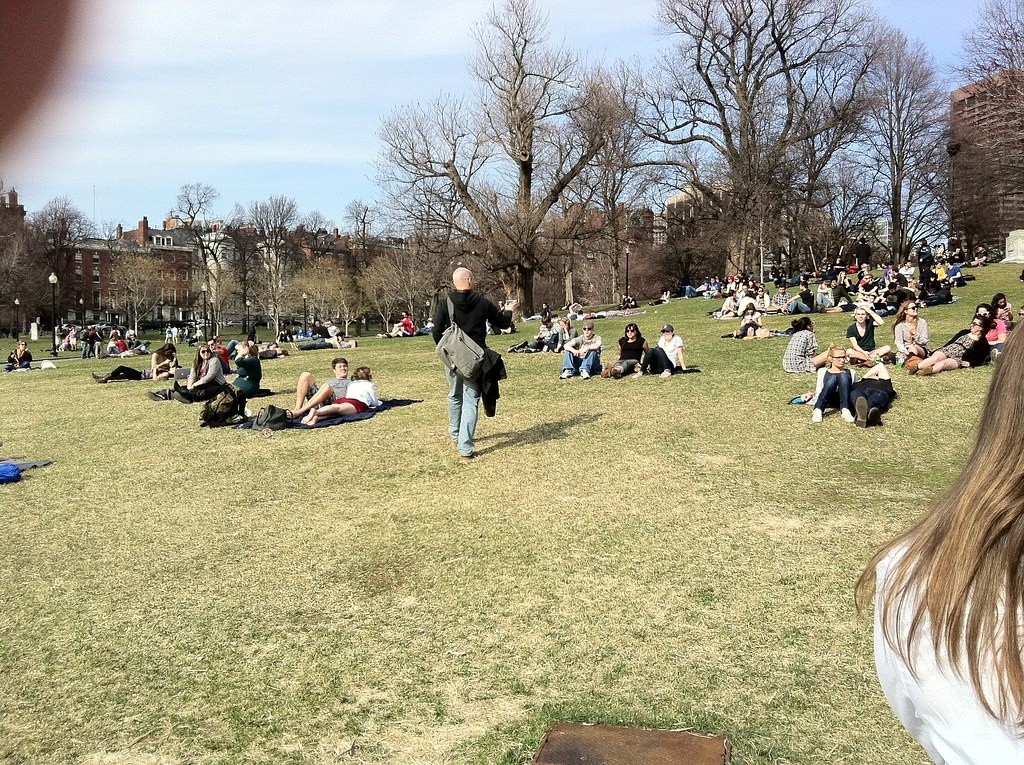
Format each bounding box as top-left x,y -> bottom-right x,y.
48,272 -> 59,356
301,292 -> 308,337
79,298 -> 84,330
200,282 -> 208,343
426,299 -> 431,324
245,299 -> 252,334
159,300 -> 165,335
14,298 -> 20,342
625,245 -> 631,297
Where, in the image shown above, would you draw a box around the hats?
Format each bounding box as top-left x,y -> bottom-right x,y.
660,325 -> 673,332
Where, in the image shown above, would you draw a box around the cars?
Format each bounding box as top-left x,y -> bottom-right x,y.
331,317 -> 382,325
55,320 -> 127,338
282,319 -> 302,328
137,317 -> 274,331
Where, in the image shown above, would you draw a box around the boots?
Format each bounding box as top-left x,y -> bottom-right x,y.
92,372 -> 112,383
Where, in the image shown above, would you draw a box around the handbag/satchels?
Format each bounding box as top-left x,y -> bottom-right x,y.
436,322 -> 484,380
252,405 -> 293,429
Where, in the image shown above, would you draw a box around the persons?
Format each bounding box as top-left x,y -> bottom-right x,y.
254,358 -> 385,426
892,292 -> 928,374
618,292 -> 639,310
782,317 -> 832,374
648,291 -> 671,305
601,323 -> 691,380
684,242 -> 993,340
857,315 -> 1024,764
146,339 -> 289,404
383,312 -> 435,338
54,323 -> 152,358
164,323 -> 204,344
429,266 -> 516,460
908,294 -> 1018,376
525,315 -> 579,354
3,340 -> 33,372
843,307 -> 892,364
277,320 -> 358,350
805,344 -> 896,429
560,321 -> 604,380
93,344 -> 179,383
521,301 -> 584,323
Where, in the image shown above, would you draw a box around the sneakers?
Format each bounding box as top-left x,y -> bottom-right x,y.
813,408 -> 823,422
558,363 -> 671,380
842,409 -> 854,422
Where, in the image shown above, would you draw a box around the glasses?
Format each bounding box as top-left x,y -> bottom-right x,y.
208,343 -> 215,346
833,356 -> 846,360
976,311 -> 989,316
663,331 -> 669,333
201,349 -> 210,353
582,328 -> 592,330
1018,313 -> 1024,316
626,329 -> 635,332
971,322 -> 981,327
907,306 -> 918,310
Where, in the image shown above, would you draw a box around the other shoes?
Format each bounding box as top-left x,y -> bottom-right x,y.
148,381 -> 204,404
856,397 -> 868,428
461,456 -> 472,460
909,363 -> 918,375
991,349 -> 1001,365
867,407 -> 880,425
453,441 -> 458,445
919,367 -> 933,376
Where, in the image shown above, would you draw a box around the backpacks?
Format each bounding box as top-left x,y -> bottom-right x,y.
201,383 -> 243,425
